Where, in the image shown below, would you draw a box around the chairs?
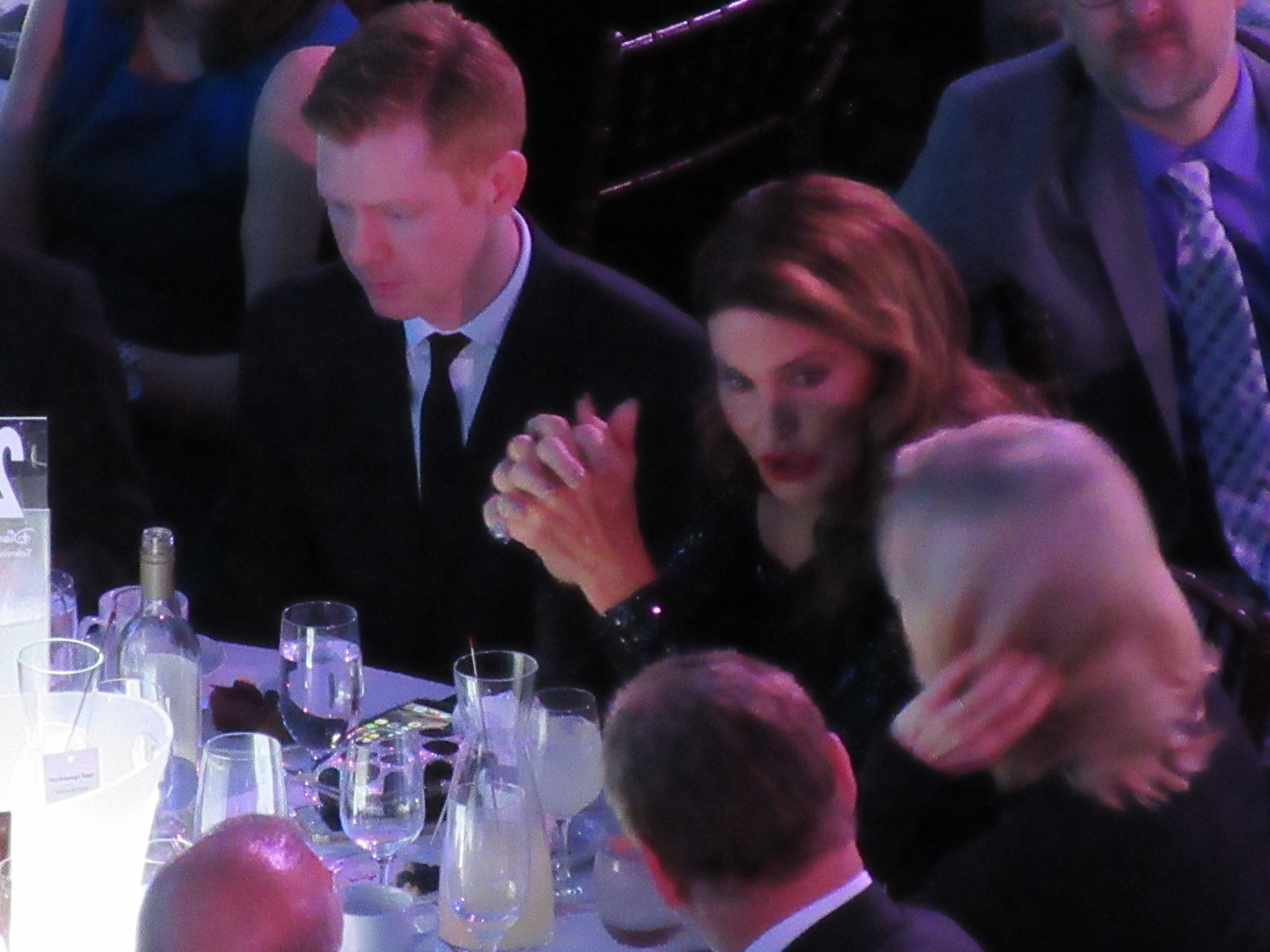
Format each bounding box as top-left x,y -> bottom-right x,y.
1163,562 -> 1270,778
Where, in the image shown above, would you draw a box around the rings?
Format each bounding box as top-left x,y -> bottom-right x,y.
490,522 -> 511,544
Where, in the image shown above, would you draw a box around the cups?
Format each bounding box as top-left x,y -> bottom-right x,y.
13,638 -> 105,692
81,583 -> 188,677
94,678 -> 176,802
50,568 -> 77,640
596,837 -> 684,948
194,732 -> 287,849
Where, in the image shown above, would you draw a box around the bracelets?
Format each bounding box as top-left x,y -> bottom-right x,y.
602,577 -> 669,632
114,340 -> 145,404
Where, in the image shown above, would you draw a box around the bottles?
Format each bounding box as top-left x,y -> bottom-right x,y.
118,527 -> 202,812
438,651 -> 556,950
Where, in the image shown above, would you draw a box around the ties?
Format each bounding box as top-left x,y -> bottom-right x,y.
1157,158 -> 1270,591
418,331 -> 474,507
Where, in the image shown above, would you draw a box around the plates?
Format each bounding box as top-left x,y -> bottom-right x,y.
195,635 -> 227,676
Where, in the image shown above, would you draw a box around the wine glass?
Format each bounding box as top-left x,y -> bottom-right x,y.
339,725 -> 438,936
527,686 -> 606,913
279,600 -> 363,841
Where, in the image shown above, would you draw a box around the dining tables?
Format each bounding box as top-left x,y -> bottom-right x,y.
0,613 -> 724,951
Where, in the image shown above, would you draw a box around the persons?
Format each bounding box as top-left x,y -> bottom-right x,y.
493,175 -> 1044,729
229,4 -> 754,696
131,811 -> 348,952
834,406 -> 1270,950
597,641 -> 973,950
0,0 -> 374,574
893,0 -> 1267,627
